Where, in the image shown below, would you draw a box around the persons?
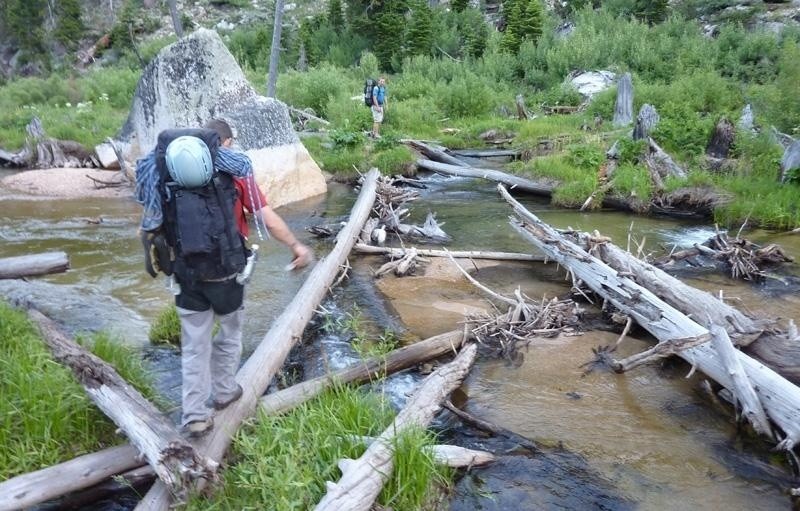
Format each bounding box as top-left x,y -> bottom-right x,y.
170,118 -> 314,437
371,78 -> 388,140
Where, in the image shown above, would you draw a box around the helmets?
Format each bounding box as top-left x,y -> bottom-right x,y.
165,137 -> 213,188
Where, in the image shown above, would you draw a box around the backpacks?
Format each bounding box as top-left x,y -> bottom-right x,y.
364,78 -> 380,107
148,128 -> 256,282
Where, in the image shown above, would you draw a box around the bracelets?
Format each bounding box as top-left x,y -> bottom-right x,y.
291,242 -> 298,248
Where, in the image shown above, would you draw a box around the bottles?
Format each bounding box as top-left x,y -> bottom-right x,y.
237,243 -> 260,285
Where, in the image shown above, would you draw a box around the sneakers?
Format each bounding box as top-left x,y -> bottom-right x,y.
212,384 -> 243,409
373,131 -> 382,138
189,415 -> 214,436
